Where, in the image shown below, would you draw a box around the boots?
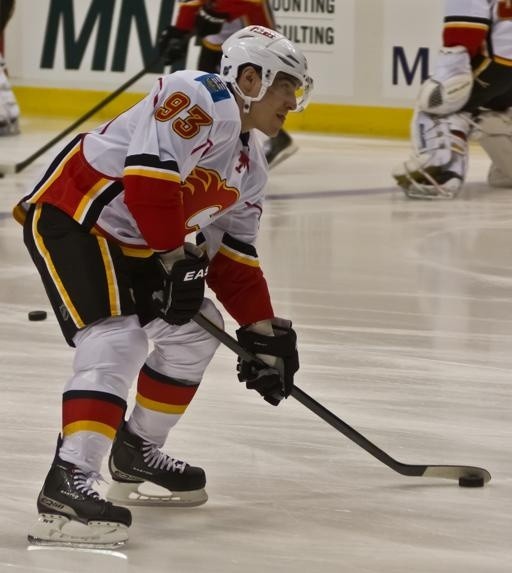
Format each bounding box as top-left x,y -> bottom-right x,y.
265,129 -> 291,163
37,433 -> 132,527
409,171 -> 463,193
110,421 -> 206,491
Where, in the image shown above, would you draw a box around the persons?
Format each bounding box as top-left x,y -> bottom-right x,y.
11,25 -> 315,529
145,0 -> 294,162
395,0 -> 512,192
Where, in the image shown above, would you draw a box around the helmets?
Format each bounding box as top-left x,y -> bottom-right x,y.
220,25 -> 313,113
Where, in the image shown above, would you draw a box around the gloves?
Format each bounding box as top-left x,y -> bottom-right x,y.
157,26 -> 189,64
236,318 -> 299,405
152,243 -> 209,326
193,5 -> 226,34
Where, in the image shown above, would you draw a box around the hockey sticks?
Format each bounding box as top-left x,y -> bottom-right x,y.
0,62 -> 150,175
151,290 -> 491,482
